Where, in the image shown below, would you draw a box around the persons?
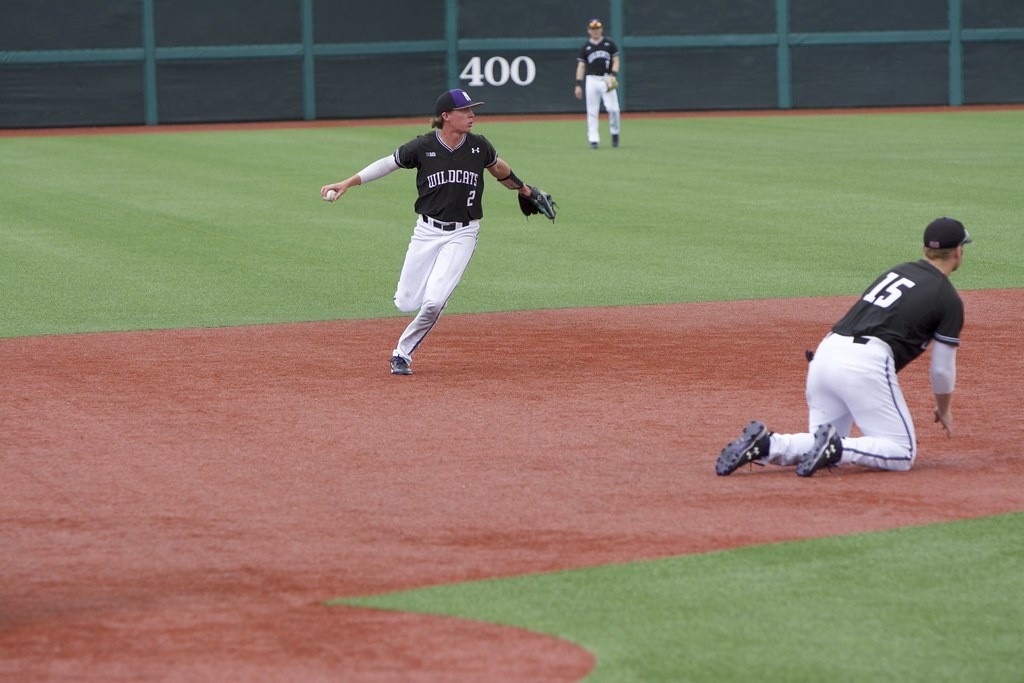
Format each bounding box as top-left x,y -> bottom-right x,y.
322,88 -> 556,375
715,216 -> 973,477
575,18 -> 621,149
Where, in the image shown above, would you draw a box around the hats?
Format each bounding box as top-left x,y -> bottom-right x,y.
434,88 -> 485,114
587,17 -> 605,28
923,217 -> 973,249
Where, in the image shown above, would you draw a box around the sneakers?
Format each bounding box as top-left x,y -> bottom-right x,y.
715,419 -> 771,476
795,422 -> 843,477
389,355 -> 413,375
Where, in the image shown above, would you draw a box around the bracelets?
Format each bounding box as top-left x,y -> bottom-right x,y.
611,72 -> 617,77
575,80 -> 582,86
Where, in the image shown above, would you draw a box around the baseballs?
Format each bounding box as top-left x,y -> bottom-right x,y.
325,190 -> 337,202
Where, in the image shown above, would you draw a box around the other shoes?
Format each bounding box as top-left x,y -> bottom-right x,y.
591,142 -> 598,149
612,135 -> 619,146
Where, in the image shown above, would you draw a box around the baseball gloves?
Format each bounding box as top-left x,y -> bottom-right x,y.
600,75 -> 619,93
518,183 -> 560,225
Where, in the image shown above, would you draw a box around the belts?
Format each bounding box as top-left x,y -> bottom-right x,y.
853,336 -> 869,344
422,214 -> 469,231
